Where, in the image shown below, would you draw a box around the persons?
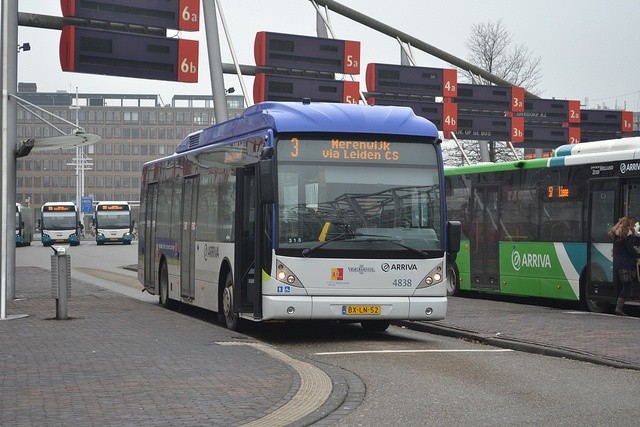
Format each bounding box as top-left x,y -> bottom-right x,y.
607,217 -> 640,315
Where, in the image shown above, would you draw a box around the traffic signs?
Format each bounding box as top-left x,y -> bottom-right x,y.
81,198 -> 92,211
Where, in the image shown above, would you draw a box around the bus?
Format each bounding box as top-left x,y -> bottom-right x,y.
138,98 -> 446,330
38,201 -> 84,247
93,200 -> 135,245
15,202 -> 33,246
411,149 -> 640,314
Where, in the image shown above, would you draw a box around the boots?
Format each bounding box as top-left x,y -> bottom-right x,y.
615,297 -> 626,316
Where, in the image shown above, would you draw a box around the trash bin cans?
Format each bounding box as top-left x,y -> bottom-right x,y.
50,243 -> 72,320
